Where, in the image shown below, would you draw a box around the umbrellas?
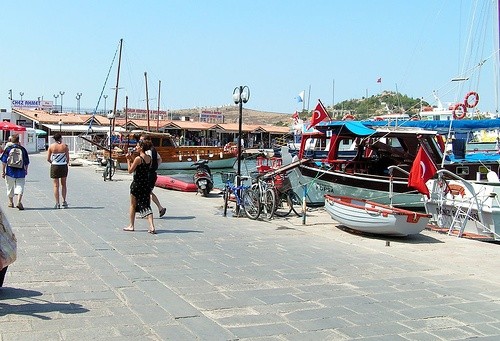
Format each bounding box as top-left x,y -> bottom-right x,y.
0,120 -> 26,145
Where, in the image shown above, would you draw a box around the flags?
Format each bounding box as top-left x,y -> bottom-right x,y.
407,145 -> 437,199
293,91 -> 304,103
306,103 -> 326,130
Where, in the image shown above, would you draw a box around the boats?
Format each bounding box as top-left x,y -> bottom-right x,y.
77,0 -> 500,242
319,193 -> 431,237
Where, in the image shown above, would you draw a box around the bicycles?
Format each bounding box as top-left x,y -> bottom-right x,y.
100,158 -> 116,181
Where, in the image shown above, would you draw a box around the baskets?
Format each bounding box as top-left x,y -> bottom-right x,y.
222,173 -> 235,183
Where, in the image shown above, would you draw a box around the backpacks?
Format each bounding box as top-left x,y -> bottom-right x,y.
139,155 -> 157,188
7,146 -> 24,168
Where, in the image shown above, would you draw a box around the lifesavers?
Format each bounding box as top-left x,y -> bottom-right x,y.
451,103 -> 467,120
373,117 -> 383,121
341,114 -> 356,121
443,184 -> 467,199
463,91 -> 480,108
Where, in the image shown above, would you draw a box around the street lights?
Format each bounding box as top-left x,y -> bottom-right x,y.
232,84 -> 251,218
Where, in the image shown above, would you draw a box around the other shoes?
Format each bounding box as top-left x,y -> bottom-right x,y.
55,204 -> 60,208
8,203 -> 14,207
16,203 -> 24,210
159,208 -> 166,217
62,201 -> 67,206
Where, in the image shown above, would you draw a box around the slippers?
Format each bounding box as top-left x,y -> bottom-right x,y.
148,230 -> 157,234
124,227 -> 134,230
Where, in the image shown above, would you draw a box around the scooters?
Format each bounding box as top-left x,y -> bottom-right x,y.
187,157 -> 214,197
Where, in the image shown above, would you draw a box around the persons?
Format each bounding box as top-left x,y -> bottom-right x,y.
0,134 -> 29,210
140,134 -> 166,217
0,206 -> 17,288
124,139 -> 155,233
46,133 -> 69,209
